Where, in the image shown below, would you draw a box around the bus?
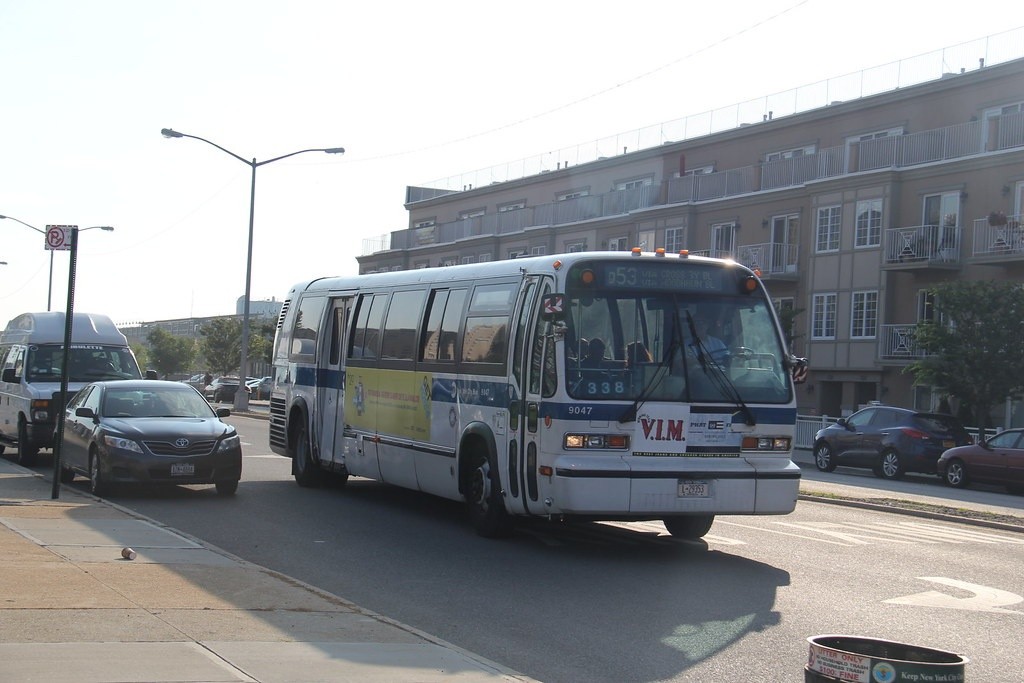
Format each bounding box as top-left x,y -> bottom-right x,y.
269,247 -> 811,537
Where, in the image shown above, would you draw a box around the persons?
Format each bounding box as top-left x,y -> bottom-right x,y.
677,313 -> 729,364
204,372 -> 213,387
568,337 -> 611,368
626,341 -> 653,370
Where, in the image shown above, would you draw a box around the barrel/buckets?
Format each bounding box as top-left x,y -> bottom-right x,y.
804,634 -> 970,683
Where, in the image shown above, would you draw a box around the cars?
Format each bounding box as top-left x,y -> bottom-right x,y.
203,377 -> 252,403
52,380 -> 242,495
936,428 -> 1024,488
813,406 -> 974,480
246,376 -> 272,401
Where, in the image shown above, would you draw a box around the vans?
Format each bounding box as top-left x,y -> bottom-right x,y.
0,312 -> 158,467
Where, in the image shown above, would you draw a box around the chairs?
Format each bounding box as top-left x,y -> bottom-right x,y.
105,397 -> 130,417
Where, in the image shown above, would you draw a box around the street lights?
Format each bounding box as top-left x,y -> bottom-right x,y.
0,216 -> 114,311
162,128 -> 344,411
